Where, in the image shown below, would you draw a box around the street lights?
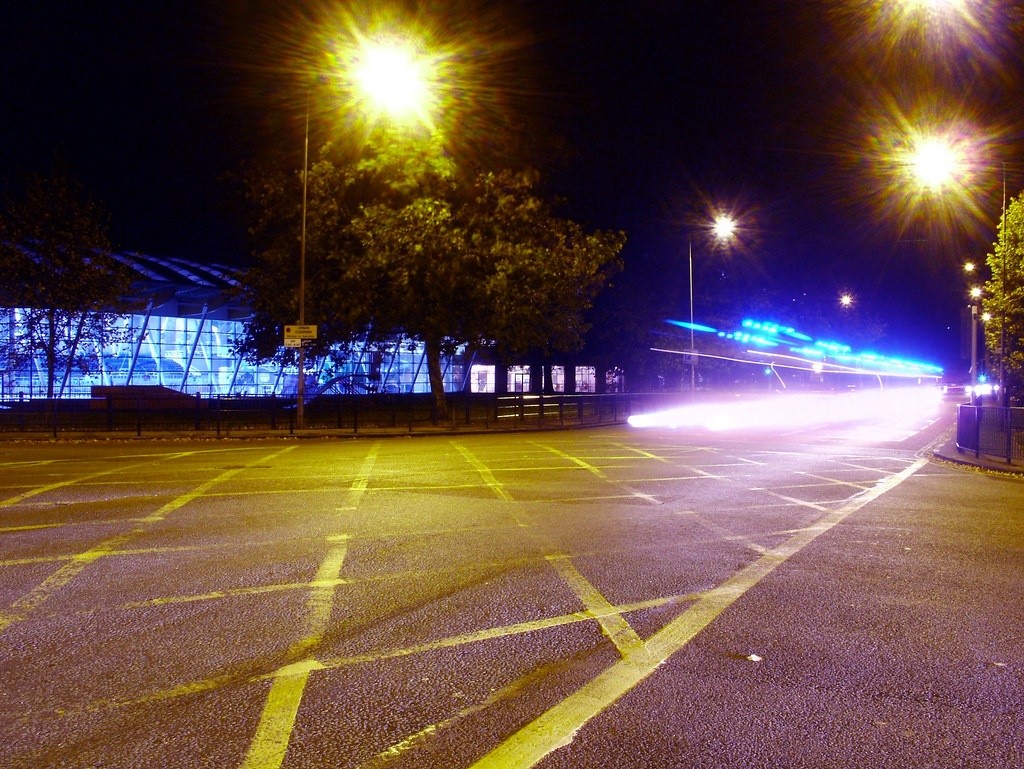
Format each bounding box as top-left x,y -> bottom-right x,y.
910,141 -> 1009,429
688,217 -> 733,403
294,40 -> 421,428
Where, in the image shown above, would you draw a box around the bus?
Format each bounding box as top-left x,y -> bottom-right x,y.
935,356 -> 983,405
